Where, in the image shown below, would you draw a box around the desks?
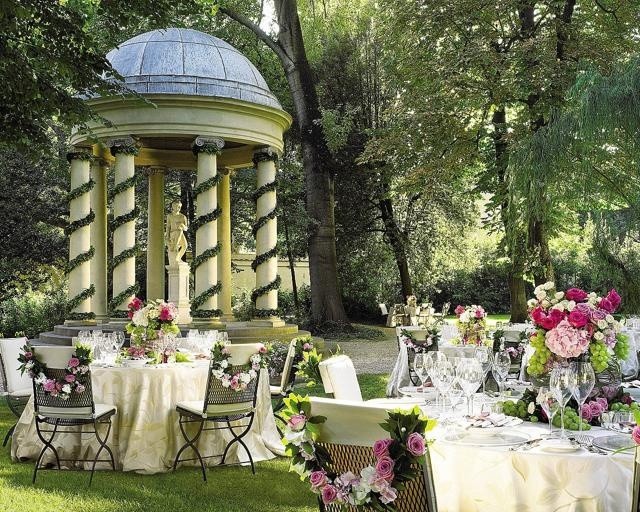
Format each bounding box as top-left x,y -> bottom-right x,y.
363,390 -> 640,512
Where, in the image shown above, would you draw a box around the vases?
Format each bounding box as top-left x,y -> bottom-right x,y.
129,328 -> 183,355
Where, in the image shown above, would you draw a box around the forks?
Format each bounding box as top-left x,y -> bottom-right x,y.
579,434 -> 606,455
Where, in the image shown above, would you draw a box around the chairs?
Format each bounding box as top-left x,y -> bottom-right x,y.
301,396 -> 438,512
268,334 -> 313,440
375,298 -> 640,398
18,343 -> 119,489
0,336 -> 38,446
319,355 -> 363,406
170,342 -> 267,483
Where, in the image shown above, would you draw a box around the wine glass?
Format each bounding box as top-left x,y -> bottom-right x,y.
539,362 -> 595,440
76,328 -> 231,367
413,345 -> 514,421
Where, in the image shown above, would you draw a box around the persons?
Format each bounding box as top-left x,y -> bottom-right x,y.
165,200 -> 188,260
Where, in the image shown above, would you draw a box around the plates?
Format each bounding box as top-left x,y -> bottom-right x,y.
593,434 -> 635,455
539,446 -> 579,452
441,426 -> 531,446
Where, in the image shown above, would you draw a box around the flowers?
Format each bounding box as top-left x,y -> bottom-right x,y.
206,340 -> 268,393
14,340 -> 95,401
523,276 -> 626,360
124,295 -> 179,345
123,345 -> 147,358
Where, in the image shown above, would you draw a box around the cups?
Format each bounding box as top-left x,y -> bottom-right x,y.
599,411 -> 635,433
